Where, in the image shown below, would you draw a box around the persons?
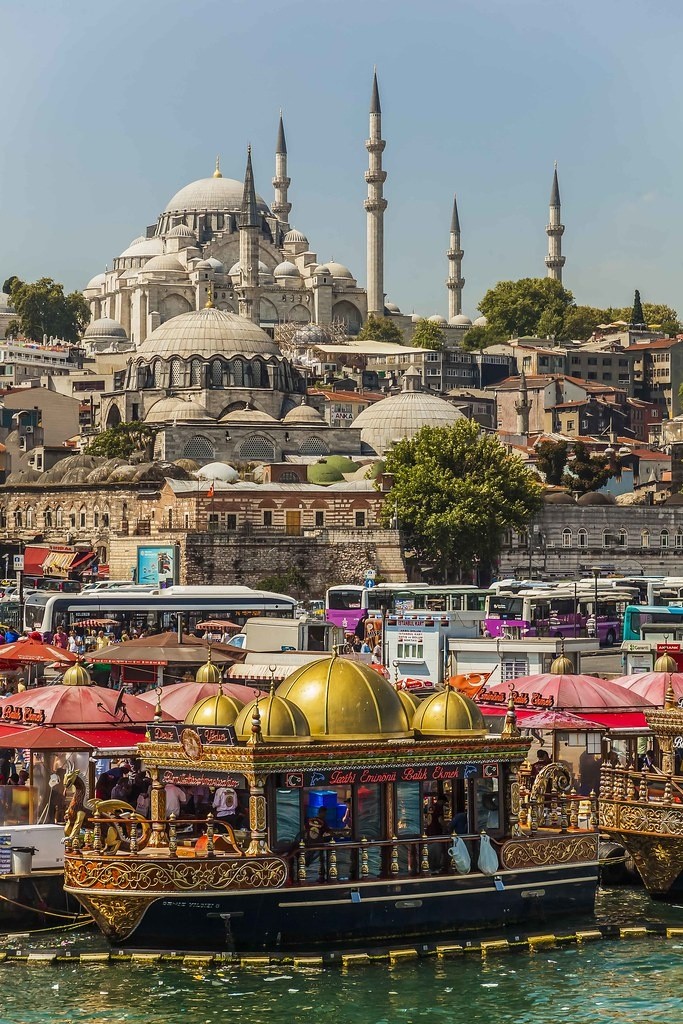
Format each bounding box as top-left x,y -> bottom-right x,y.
121,614 -> 178,642
0,674 -> 27,697
0,623 -> 43,645
426,794 -> 468,837
52,626 -> 115,653
353,637 -> 382,664
305,797 -> 353,840
529,750 -> 558,829
482,627 -> 491,639
585,613 -> 598,638
94,768 -> 237,833
0,750 -> 30,785
202,630 -> 230,644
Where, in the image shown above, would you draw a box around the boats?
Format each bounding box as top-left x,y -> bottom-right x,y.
598,675 -> 683,895
61,634 -> 601,952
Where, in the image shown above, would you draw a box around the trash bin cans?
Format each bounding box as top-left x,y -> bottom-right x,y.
10,847 -> 33,874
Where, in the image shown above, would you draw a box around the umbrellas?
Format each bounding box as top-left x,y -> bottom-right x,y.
516,712 -> 608,765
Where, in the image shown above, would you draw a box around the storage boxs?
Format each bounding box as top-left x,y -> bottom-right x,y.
305,789 -> 352,841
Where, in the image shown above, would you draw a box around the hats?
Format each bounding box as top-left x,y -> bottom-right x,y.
9,774 -> 19,779
124,764 -> 133,773
48,774 -> 60,787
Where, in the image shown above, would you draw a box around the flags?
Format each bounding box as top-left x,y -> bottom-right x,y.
206,483 -> 214,497
448,671 -> 492,701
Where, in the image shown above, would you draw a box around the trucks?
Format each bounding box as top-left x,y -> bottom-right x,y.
226,618 -> 345,651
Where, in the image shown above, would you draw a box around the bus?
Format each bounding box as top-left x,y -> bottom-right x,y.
482,590 -> 640,648
621,605 -> 683,667
485,576 -> 683,615
326,583 -> 496,638
23,585 -> 299,634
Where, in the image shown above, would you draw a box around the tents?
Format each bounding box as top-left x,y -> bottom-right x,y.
68,619 -> 120,627
196,619 -> 243,630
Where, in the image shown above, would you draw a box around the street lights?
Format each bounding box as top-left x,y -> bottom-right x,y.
380,602 -> 389,667
591,566 -> 602,638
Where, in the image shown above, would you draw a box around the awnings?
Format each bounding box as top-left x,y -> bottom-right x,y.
23,546 -> 96,577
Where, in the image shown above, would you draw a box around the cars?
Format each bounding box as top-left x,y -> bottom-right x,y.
0,577 -> 136,603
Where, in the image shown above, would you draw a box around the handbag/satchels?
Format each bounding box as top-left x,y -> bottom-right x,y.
136,795 -> 150,817
447,836 -> 471,875
478,834 -> 498,876
